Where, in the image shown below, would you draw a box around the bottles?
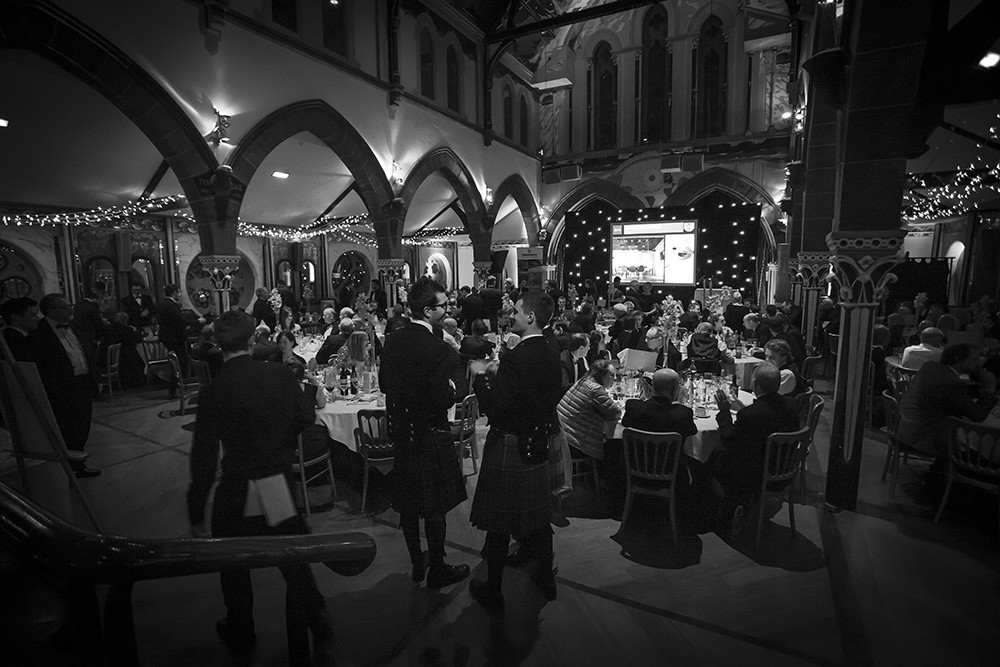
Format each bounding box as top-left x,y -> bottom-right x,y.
725,329 -> 745,359
611,367 -> 738,408
340,365 -> 357,396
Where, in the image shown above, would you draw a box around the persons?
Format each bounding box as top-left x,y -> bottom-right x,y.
1,275 -> 806,538
378,279 -> 471,588
471,289 -> 564,598
187,311 -> 326,656
821,299 -> 999,511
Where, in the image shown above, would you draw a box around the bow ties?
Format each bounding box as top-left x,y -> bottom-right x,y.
56,324 -> 70,328
135,296 -> 141,298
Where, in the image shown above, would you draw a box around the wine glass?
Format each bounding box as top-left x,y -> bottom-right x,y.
294,332 -> 323,356
325,372 -> 336,403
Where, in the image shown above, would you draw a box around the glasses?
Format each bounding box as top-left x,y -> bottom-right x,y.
646,337 -> 658,340
427,300 -> 449,309
52,305 -> 74,310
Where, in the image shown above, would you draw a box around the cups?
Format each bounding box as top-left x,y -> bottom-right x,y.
695,407 -> 706,416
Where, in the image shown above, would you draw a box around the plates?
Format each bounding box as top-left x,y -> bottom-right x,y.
696,414 -> 711,418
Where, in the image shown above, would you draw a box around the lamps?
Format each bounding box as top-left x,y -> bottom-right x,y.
392,160 -> 404,186
486,187 -> 494,206
213,110 -> 231,144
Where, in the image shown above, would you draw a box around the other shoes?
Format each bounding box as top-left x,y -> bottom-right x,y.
71,461 -> 101,478
312,611 -> 333,643
469,578 -> 504,612
426,564 -> 471,587
217,615 -> 259,654
531,570 -> 556,600
412,549 -> 428,578
724,503 -> 742,537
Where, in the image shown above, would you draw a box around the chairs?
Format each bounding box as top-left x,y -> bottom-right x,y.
66,271 -> 1000,566
